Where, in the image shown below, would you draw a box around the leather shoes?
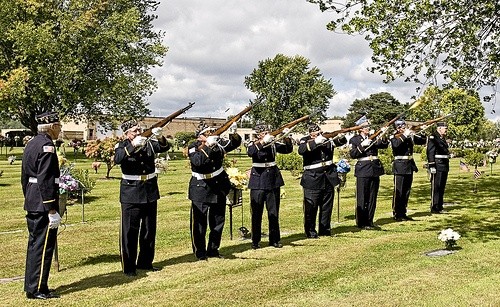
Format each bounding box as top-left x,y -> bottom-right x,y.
126,266 -> 162,276
27,291 -> 59,300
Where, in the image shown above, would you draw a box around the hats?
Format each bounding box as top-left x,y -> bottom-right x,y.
309,125 -> 323,133
36,111 -> 59,124
394,119 -> 447,126
252,125 -> 268,134
121,119 -> 138,132
355,115 -> 368,125
196,121 -> 215,136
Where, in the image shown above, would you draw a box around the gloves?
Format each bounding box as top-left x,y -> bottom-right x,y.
403,122 -> 434,138
131,126 -> 163,147
48,212 -> 61,229
315,131 -> 345,144
361,127 -> 389,147
263,128 -> 290,144
430,167 -> 437,174
206,122 -> 237,146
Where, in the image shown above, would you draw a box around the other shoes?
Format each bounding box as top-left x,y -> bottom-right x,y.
252,243 -> 282,251
307,233 -> 331,238
199,254 -> 224,261
393,216 -> 413,222
431,209 -> 448,214
357,224 -> 380,230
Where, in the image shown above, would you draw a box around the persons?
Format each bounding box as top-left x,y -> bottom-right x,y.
390,121 -> 429,222
114,118 -> 170,277
246,125 -> 293,249
21,111 -> 62,299
187,120 -> 242,261
426,122 -> 453,215
349,116 -> 389,230
298,124 -> 347,239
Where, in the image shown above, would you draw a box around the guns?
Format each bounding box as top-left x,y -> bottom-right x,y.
132,101 -> 196,139
367,109 -> 407,140
253,111 -> 317,145
197,96 -> 264,151
395,113 -> 451,140
307,121 -> 374,143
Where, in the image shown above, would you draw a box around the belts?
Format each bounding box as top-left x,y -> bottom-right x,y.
303,159 -> 333,170
121,172 -> 156,181
357,155 -> 450,162
252,162 -> 277,167
191,166 -> 223,180
29,177 -> 59,184
